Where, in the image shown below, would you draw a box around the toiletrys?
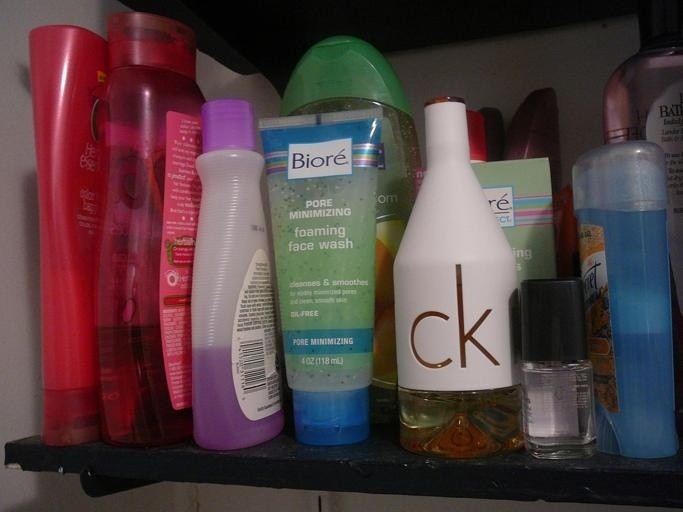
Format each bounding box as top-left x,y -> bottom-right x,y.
394,95 -> 520,460
522,279 -> 598,462
257,108 -> 384,444
